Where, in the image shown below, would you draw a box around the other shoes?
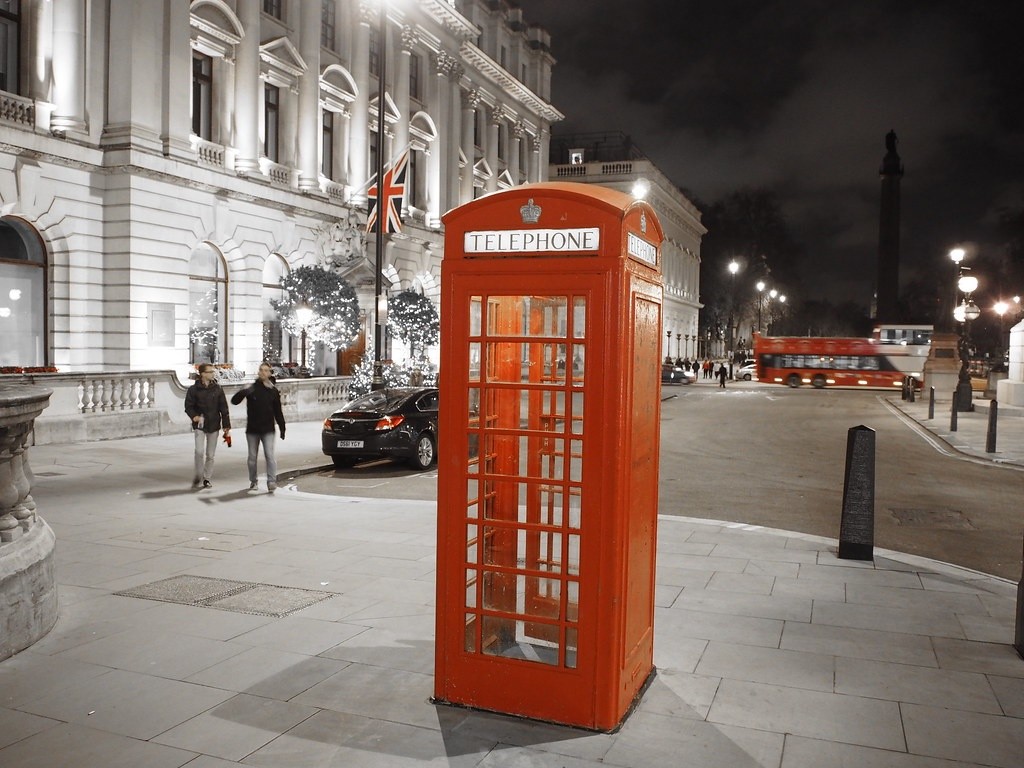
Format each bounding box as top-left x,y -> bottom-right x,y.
191,480 -> 202,491
250,482 -> 258,490
268,485 -> 275,493
203,480 -> 212,487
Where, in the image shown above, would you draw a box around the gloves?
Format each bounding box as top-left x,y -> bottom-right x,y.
280,422 -> 286,431
246,389 -> 256,401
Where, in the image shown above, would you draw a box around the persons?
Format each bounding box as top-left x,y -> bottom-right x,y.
692,359 -> 700,380
232,362 -> 286,494
707,358 -> 714,379
665,355 -> 692,373
717,363 -> 728,388
702,360 -> 709,378
184,362 -> 232,490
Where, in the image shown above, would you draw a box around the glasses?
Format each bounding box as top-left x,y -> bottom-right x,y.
205,370 -> 215,373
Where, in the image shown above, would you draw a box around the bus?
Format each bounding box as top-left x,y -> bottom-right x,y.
750,329 -> 924,388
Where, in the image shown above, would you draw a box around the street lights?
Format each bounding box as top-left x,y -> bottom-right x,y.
769,288 -> 778,335
726,259 -> 739,380
756,280 -> 766,332
949,247 -> 981,413
779,294 -> 787,335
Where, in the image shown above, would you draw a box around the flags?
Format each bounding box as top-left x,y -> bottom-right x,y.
366,146 -> 408,233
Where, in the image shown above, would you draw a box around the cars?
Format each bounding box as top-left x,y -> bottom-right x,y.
320,385 -> 480,472
661,361 -> 696,385
734,363 -> 758,381
